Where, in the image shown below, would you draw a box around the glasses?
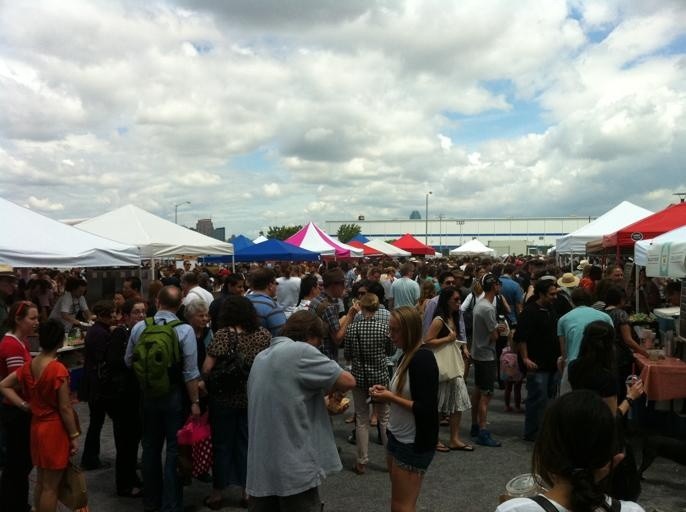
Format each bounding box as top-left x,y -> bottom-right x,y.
271,282 -> 279,285
356,291 -> 368,297
449,297 -> 460,304
443,280 -> 456,286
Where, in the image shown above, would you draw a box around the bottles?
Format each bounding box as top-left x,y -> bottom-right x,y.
68,328 -> 72,345
498,315 -> 505,325
74,329 -> 81,345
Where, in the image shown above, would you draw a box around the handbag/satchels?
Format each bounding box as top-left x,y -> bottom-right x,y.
212,326 -> 249,395
497,296 -> 510,336
58,450 -> 88,509
424,317 -> 464,382
90,328 -> 140,406
462,293 -> 477,337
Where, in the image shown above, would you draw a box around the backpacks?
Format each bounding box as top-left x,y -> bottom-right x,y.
606,397 -> 654,501
501,351 -> 524,382
134,317 -> 187,399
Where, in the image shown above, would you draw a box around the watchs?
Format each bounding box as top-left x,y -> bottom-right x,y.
625,396 -> 635,406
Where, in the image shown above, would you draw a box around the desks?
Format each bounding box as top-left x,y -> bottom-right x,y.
631,350 -> 686,473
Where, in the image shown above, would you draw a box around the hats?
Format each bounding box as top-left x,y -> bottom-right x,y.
320,270 -> 349,286
577,260 -> 589,269
557,274 -> 580,287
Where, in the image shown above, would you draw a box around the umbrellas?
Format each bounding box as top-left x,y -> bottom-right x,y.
616,201 -> 686,247
390,234 -> 435,254
252,236 -> 268,244
363,237 -> 412,256
635,223 -> 686,277
556,200 -> 655,255
344,239 -> 382,256
232,239 -> 320,260
284,221 -> 363,257
0,197 -> 141,267
603,203 -> 675,250
72,203 -> 235,258
203,235 -> 256,260
349,234 -> 369,243
448,238 -> 494,256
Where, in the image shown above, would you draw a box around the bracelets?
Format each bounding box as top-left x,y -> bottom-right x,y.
69,432 -> 80,440
18,400 -> 26,409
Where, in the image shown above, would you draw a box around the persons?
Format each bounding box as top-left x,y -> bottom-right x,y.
569,321 -> 645,422
307,269 -> 361,360
602,286 -> 650,429
114,297 -> 147,343
423,272 -> 470,357
39,275 -> 55,346
388,264 -> 419,308
126,287 -> 201,511
245,268 -> 287,337
498,328 -> 524,411
494,390 -> 647,512
274,261 -> 301,309
382,268 -> 399,303
0,318 -> 80,512
422,288 -> 474,451
497,252 -> 557,284
632,264 -> 683,304
200,295 -> 272,505
352,267 -> 385,299
122,278 -> 145,298
558,289 -> 614,396
340,262 -> 356,290
557,258 -> 626,310
1,267 -> 85,294
48,279 -> 99,335
179,272 -> 214,315
81,298 -> 125,469
458,272 -> 491,379
246,309 -> 356,512
223,273 -> 246,296
344,294 -> 397,474
141,259 -> 193,287
300,257 -> 409,271
0,295 -> 38,512
471,273 -> 507,446
183,300 -> 215,364
409,255 -> 496,272
292,274 -> 322,314
368,307 -> 439,511
518,280 -> 558,441
112,291 -> 130,308
499,265 -> 524,336
